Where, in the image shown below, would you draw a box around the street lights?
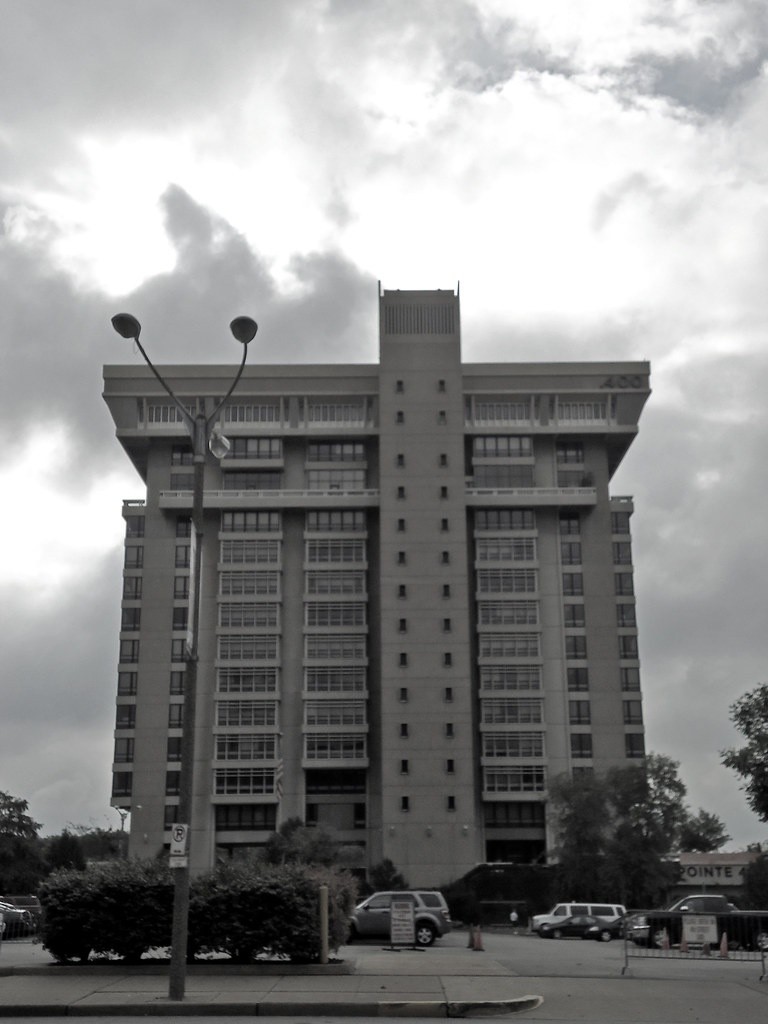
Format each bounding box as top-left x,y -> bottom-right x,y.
110,310 -> 260,1002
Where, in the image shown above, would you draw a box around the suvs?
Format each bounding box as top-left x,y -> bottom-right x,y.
343,891 -> 455,948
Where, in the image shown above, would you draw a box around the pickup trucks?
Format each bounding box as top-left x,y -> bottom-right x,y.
622,894 -> 768,952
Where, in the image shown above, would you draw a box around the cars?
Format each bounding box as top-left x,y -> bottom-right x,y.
538,909 -> 658,943
0,896 -> 43,936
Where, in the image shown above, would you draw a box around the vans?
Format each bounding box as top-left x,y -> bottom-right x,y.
529,902 -> 628,934
